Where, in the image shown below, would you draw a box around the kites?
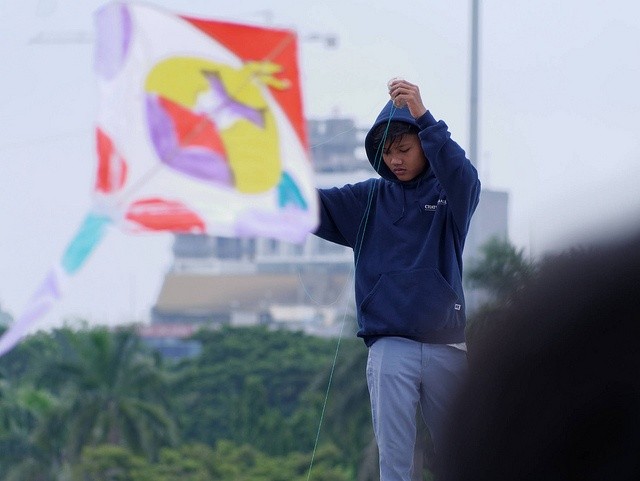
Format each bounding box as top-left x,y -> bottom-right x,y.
60,2 -> 321,276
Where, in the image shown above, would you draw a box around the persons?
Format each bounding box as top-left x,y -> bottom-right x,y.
306,80 -> 482,477
